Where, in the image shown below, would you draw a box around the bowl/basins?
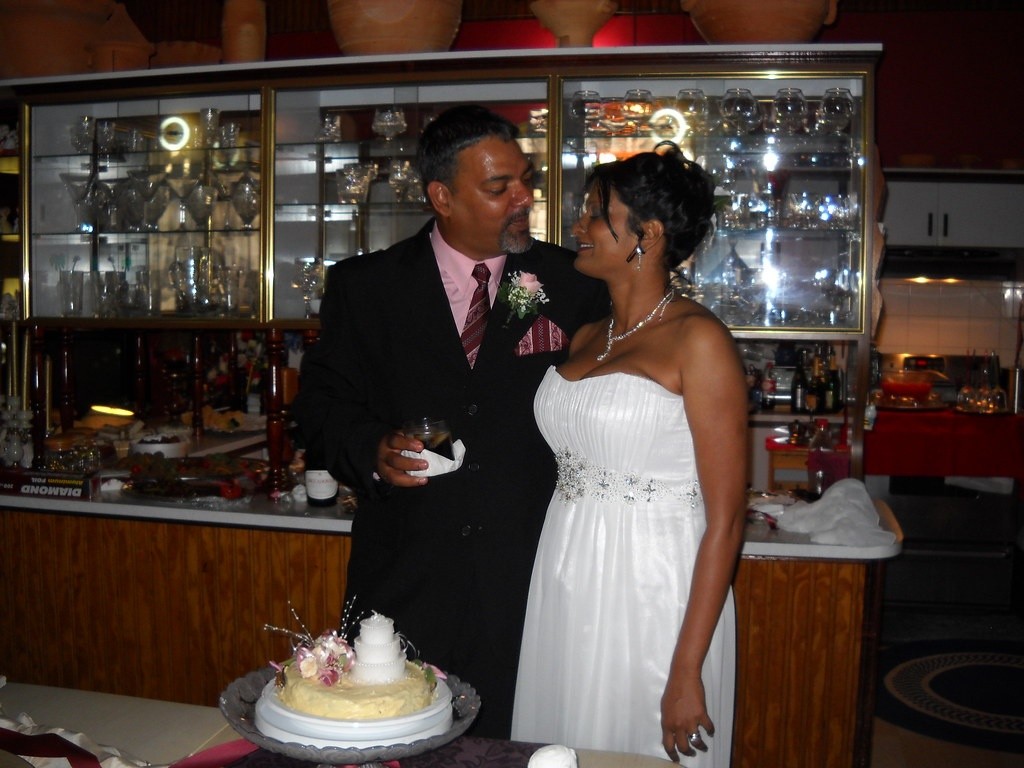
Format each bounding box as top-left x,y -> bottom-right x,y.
132,436 -> 187,458
82,40 -> 155,73
326,0 -> 461,56
681,0 -> 836,45
530,0 -> 618,48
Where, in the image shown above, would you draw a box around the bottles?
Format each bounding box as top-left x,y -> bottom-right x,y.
807,418 -> 834,493
223,0 -> 266,63
763,362 -> 776,408
792,344 -> 845,414
304,448 -> 338,507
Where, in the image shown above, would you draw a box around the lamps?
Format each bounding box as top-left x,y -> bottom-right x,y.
0,307 -> 54,471
89,351 -> 140,418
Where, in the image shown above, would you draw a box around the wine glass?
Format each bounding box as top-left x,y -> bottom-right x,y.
274,106 -> 549,315
560,89 -> 862,330
36,107 -> 262,319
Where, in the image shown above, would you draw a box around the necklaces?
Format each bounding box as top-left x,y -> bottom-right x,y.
597,288 -> 675,361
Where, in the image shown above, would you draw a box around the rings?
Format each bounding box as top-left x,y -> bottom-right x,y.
688,733 -> 700,741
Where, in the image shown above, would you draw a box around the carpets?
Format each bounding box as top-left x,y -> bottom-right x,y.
873,638 -> 1024,754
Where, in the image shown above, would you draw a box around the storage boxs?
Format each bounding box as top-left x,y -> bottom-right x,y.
767,449 -> 852,497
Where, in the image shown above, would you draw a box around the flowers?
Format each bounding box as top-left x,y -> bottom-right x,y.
496,270 -> 550,328
298,653 -> 350,687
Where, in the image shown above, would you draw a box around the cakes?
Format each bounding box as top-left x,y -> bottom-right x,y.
255,613 -> 453,748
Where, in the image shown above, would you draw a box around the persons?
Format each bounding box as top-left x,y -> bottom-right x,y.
510,140 -> 747,768
293,105 -> 611,740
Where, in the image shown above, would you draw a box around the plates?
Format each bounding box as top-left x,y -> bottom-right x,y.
220,659 -> 481,768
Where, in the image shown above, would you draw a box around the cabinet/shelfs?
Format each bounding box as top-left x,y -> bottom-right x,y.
878,167 -> 1024,282
0,42 -> 903,768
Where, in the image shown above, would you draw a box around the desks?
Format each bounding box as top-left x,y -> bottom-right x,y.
168,736 -> 687,768
863,404 -> 1024,496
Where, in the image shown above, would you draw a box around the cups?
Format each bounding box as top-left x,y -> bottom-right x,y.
402,416 -> 456,462
957,386 -> 1007,413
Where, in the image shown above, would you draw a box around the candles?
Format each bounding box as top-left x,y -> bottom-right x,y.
984,350 -> 989,369
